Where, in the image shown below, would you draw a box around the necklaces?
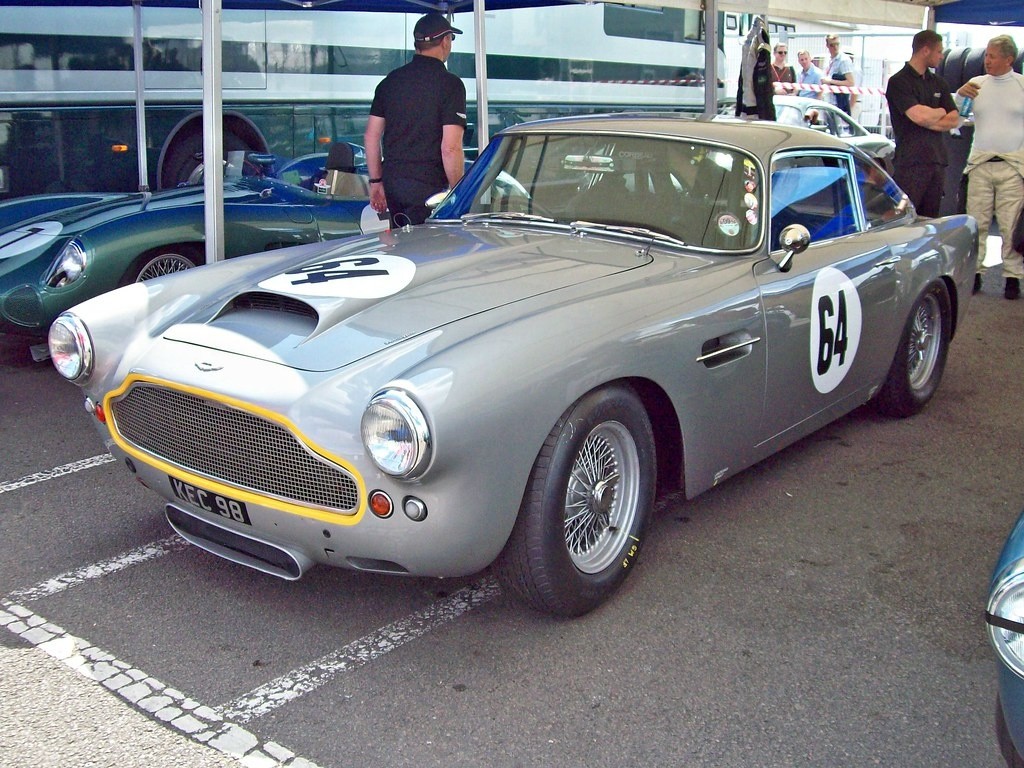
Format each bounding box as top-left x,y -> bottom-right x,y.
772,64 -> 787,82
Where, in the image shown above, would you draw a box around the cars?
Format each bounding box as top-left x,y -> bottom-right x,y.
47,115 -> 979,617
723,96 -> 895,170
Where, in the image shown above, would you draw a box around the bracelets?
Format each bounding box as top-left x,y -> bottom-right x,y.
367,176 -> 382,183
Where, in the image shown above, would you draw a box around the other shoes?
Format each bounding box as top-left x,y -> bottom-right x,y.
1004,277 -> 1021,300
972,274 -> 982,296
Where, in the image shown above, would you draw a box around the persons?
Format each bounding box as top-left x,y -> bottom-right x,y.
790,50 -> 824,101
769,42 -> 798,82
953,34 -> 1023,300
818,33 -> 855,116
884,29 -> 959,219
362,11 -> 468,233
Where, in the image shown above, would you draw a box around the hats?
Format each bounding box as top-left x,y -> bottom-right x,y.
413,14 -> 463,41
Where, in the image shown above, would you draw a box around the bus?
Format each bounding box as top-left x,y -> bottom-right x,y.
0,1 -> 735,191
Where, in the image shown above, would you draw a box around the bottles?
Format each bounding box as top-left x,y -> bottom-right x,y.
960,97 -> 974,116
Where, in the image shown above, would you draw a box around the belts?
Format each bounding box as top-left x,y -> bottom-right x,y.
986,156 -> 1005,162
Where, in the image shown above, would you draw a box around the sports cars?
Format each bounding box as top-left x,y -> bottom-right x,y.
0,164 -> 468,354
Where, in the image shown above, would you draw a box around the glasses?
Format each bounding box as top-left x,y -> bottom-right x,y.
829,43 -> 841,47
776,51 -> 788,54
451,33 -> 455,40
